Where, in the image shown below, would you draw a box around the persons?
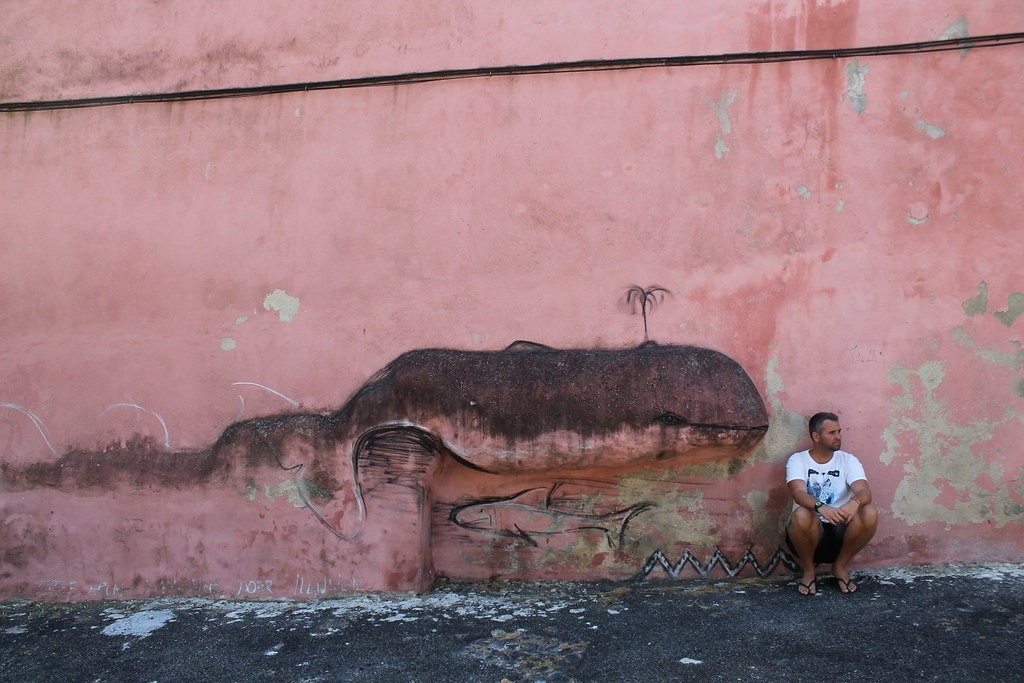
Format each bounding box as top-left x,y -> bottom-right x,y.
784,410 -> 879,597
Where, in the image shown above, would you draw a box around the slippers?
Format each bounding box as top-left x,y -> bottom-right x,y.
798,576 -> 817,596
828,577 -> 858,596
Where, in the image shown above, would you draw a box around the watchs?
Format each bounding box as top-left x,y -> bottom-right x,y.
815,501 -> 824,513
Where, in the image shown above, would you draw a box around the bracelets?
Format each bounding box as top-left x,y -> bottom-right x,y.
850,498 -> 860,508
855,497 -> 862,507
